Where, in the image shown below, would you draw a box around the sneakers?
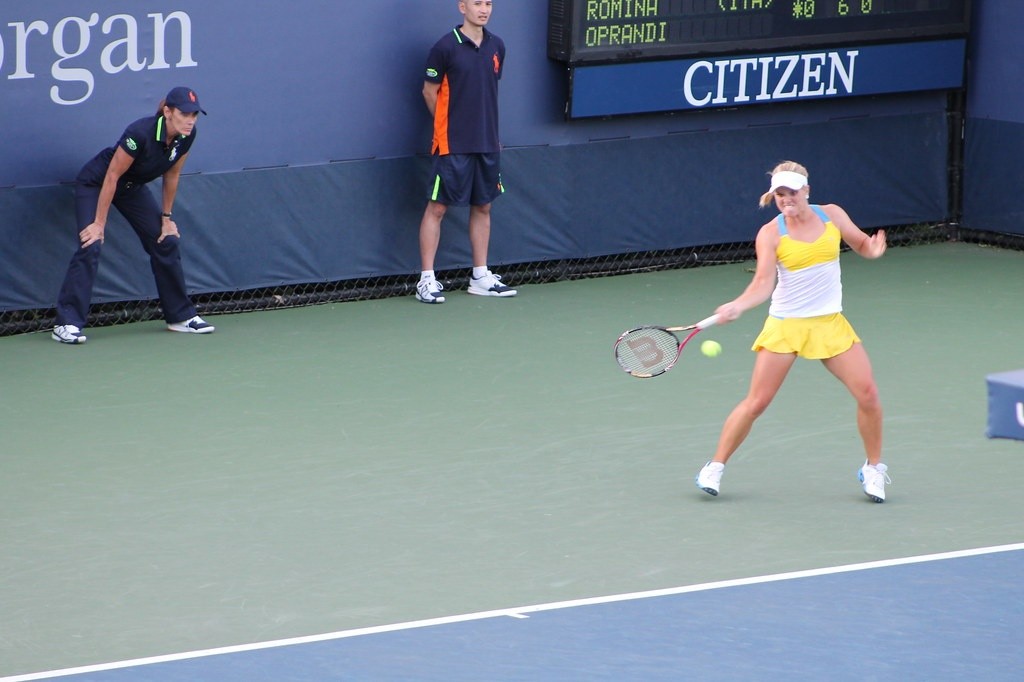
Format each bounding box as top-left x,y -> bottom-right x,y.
52,325 -> 86,343
415,275 -> 445,303
696,461 -> 725,496
467,271 -> 517,296
168,316 -> 214,333
858,458 -> 891,503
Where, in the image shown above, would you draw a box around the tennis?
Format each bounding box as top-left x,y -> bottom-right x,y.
701,339 -> 721,357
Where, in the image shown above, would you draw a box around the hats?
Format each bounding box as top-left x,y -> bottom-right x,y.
768,171 -> 807,193
165,87 -> 207,115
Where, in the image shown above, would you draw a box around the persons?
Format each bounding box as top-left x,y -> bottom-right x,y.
695,160 -> 891,504
50,87 -> 215,345
414,0 -> 519,303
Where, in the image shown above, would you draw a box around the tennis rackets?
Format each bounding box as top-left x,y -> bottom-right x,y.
614,314 -> 722,379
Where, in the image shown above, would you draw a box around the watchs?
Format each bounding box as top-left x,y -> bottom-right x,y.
160,210 -> 172,216
